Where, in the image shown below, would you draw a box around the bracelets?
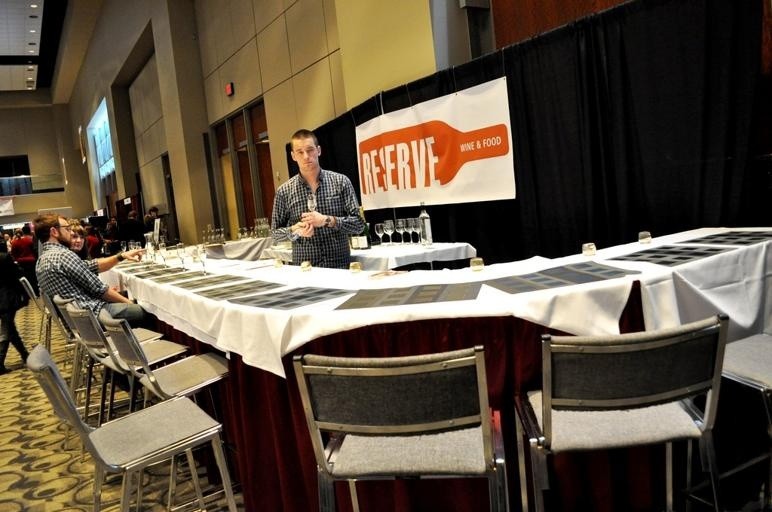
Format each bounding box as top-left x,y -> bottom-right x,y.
325,216 -> 331,227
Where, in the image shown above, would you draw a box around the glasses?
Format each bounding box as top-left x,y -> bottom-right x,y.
58,225 -> 71,232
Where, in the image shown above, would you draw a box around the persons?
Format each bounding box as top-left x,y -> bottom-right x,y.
0,239 -> 30,376
270,130 -> 366,270
0,206 -> 158,307
32,213 -> 149,338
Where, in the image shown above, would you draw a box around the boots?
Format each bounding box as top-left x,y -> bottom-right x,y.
1,337 -> 28,374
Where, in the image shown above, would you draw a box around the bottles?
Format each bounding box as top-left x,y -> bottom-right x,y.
203,224 -> 226,245
349,207 -> 371,250
418,200 -> 433,247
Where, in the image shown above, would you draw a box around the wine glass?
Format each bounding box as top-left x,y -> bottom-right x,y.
196,244 -> 208,277
236,216 -> 270,241
374,218 -> 426,248
176,243 -> 188,272
120,231 -> 168,268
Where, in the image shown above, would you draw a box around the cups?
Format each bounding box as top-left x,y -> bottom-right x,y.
300,260 -> 312,273
638,230 -> 651,247
349,262 -> 360,276
273,258 -> 283,271
469,258 -> 484,274
581,243 -> 597,260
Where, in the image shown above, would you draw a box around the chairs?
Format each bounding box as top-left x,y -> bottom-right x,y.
514,313 -> 730,511
293,344 -> 512,511
686,333 -> 772,510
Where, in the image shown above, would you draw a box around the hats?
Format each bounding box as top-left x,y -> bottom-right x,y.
149,207 -> 158,215
